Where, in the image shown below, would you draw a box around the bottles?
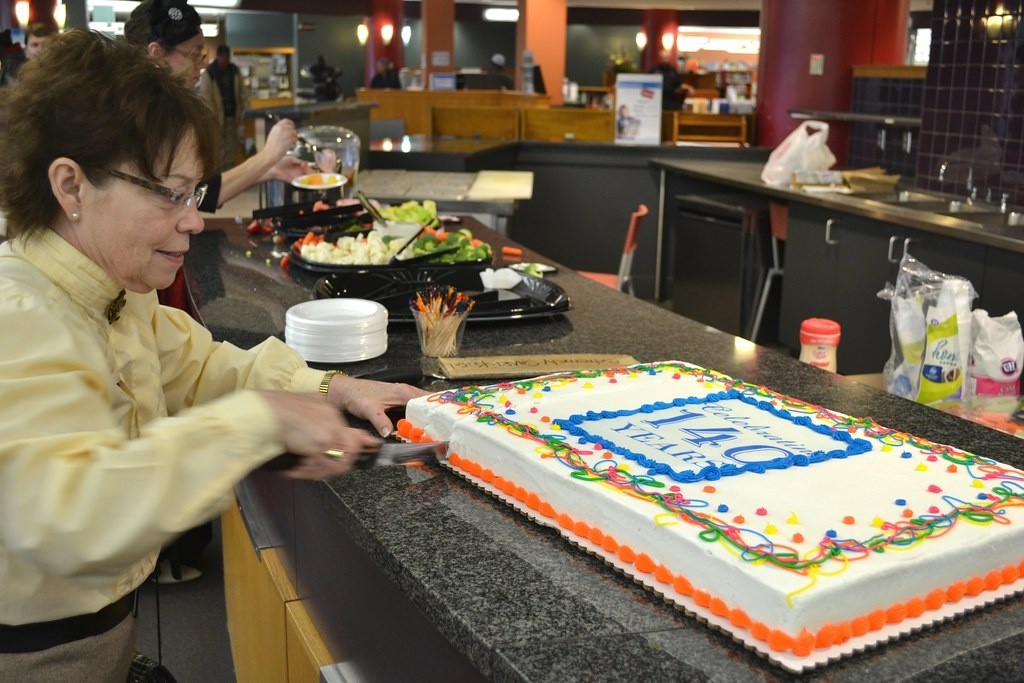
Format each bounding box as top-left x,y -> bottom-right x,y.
797,317 -> 841,374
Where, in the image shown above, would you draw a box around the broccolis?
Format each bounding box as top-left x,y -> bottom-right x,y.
300,231 -> 491,266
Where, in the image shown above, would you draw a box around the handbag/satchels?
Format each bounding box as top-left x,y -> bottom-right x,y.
761,119 -> 837,184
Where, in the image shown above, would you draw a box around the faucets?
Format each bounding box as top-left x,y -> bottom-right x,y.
1000,192 -> 1010,215
938,158 -> 979,206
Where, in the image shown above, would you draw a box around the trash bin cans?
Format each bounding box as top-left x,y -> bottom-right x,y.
671,194 -> 784,344
242,101 -> 380,169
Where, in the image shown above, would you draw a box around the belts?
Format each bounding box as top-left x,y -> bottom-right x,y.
0,588 -> 138,653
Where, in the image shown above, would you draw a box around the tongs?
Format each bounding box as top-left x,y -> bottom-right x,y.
390,218 -> 461,267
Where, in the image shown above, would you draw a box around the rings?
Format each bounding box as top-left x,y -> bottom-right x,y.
326,449 -> 344,460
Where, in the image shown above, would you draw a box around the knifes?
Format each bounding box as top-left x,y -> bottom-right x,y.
265,440 -> 449,472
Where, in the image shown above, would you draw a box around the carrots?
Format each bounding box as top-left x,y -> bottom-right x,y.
280,232 -> 336,268
418,227 -> 523,254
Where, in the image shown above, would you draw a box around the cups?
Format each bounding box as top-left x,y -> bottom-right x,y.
409,304 -> 468,357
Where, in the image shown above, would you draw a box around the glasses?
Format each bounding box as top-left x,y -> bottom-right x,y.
156,38 -> 210,64
110,168 -> 209,208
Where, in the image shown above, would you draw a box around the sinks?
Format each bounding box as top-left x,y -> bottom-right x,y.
957,211 -> 1024,226
841,190 -> 947,203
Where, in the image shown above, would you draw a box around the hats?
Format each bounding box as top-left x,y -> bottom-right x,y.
124,0 -> 202,44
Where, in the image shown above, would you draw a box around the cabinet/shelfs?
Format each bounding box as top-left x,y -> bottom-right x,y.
778,201 -> 1024,376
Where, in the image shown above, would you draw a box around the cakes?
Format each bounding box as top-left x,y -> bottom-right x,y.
396,360 -> 1024,659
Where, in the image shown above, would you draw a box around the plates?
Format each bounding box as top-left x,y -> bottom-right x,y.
291,172 -> 348,190
311,267 -> 574,323
288,233 -> 494,280
284,297 -> 388,363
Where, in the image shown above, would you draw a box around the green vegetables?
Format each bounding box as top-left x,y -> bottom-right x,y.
378,196 -> 441,228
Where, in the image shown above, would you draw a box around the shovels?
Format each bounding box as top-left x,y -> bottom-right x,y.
354,366 -> 448,384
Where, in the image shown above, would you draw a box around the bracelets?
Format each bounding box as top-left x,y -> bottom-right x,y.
320,370 -> 350,397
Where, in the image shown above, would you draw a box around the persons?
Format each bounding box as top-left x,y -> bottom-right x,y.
0,0 -> 432,683
649,47 -> 691,108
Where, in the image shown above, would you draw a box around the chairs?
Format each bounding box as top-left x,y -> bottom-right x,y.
748,199 -> 788,344
573,202 -> 648,297
